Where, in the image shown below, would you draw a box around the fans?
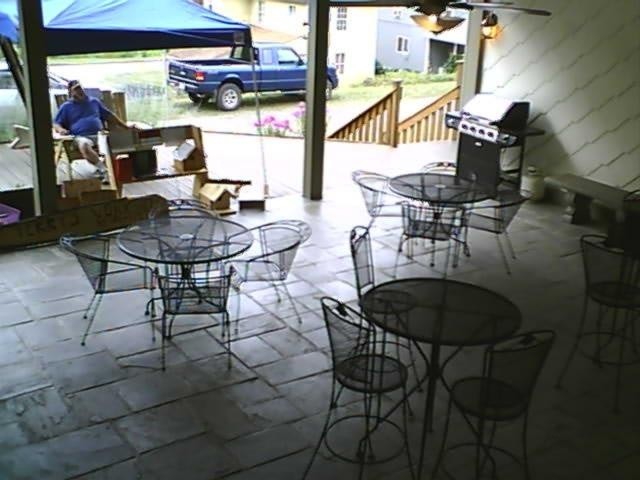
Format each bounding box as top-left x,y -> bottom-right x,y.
329,0 -> 553,35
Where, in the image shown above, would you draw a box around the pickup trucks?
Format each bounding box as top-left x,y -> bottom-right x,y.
167,41 -> 338,110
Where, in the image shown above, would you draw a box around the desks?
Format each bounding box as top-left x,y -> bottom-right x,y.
388,172 -> 493,256
357,274 -> 526,435
442,111 -> 547,200
116,215 -> 252,338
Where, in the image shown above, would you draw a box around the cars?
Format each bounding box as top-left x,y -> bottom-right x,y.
0,62 -> 124,128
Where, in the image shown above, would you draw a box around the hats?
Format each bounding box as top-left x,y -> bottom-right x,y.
68,80 -> 80,90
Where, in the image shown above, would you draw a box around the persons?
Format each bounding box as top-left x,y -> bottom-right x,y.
53,81 -> 138,185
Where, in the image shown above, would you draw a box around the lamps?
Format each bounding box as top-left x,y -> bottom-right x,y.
479,12 -> 500,41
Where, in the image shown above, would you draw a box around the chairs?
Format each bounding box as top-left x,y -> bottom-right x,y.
150,198 -> 220,233
53,90 -> 118,182
351,171 -> 405,241
554,233 -> 638,414
300,291 -> 416,479
396,201 -> 463,269
464,192 -> 534,273
61,233 -> 158,347
349,224 -> 418,346
435,329 -> 558,480
154,264 -> 239,370
620,187 -> 639,231
225,218 -> 311,323
